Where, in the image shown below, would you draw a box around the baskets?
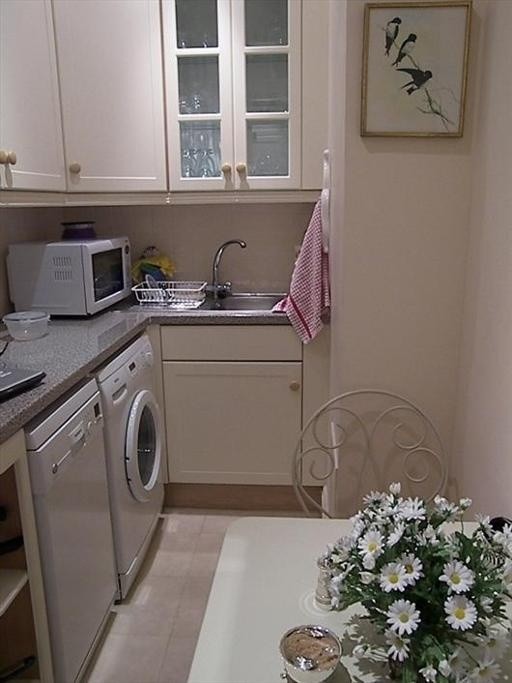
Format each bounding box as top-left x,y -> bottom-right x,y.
132,280 -> 208,304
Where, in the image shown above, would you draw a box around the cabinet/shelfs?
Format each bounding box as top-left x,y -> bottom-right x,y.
0,0 -> 328,209
159,324 -> 303,486
1,430 -> 56,682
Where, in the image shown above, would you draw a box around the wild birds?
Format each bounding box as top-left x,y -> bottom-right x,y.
384,17 -> 402,57
396,68 -> 432,95
391,34 -> 417,68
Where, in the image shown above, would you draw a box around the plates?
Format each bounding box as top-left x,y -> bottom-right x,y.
146,273 -> 163,302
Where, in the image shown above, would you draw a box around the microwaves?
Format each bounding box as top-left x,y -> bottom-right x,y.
6,234 -> 133,319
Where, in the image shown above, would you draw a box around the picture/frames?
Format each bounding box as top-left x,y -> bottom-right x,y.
360,1 -> 472,139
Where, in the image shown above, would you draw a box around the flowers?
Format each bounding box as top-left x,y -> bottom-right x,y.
317,485 -> 512,682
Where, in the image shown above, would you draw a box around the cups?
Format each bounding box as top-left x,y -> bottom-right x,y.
278,625 -> 342,683
179,88 -> 207,113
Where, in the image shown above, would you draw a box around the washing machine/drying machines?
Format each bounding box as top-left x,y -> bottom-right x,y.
87,331 -> 167,601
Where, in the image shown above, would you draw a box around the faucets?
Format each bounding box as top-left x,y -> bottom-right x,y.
211,238 -> 248,285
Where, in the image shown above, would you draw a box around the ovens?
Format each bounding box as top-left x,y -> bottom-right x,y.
22,375 -> 121,682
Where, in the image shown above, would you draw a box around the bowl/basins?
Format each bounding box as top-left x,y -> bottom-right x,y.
58,218 -> 98,242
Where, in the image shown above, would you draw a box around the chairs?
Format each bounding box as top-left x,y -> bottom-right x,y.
291,389 -> 449,518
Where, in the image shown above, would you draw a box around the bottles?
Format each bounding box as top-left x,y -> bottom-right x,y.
312,553 -> 340,613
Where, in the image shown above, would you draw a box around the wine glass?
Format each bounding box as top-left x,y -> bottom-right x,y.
183,129 -> 216,178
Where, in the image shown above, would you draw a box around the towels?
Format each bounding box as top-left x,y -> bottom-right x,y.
273,200 -> 330,345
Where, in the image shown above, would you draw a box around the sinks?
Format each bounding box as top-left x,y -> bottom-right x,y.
197,293 -> 287,312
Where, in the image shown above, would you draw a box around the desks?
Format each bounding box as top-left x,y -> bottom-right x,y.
186,516 -> 512,683
